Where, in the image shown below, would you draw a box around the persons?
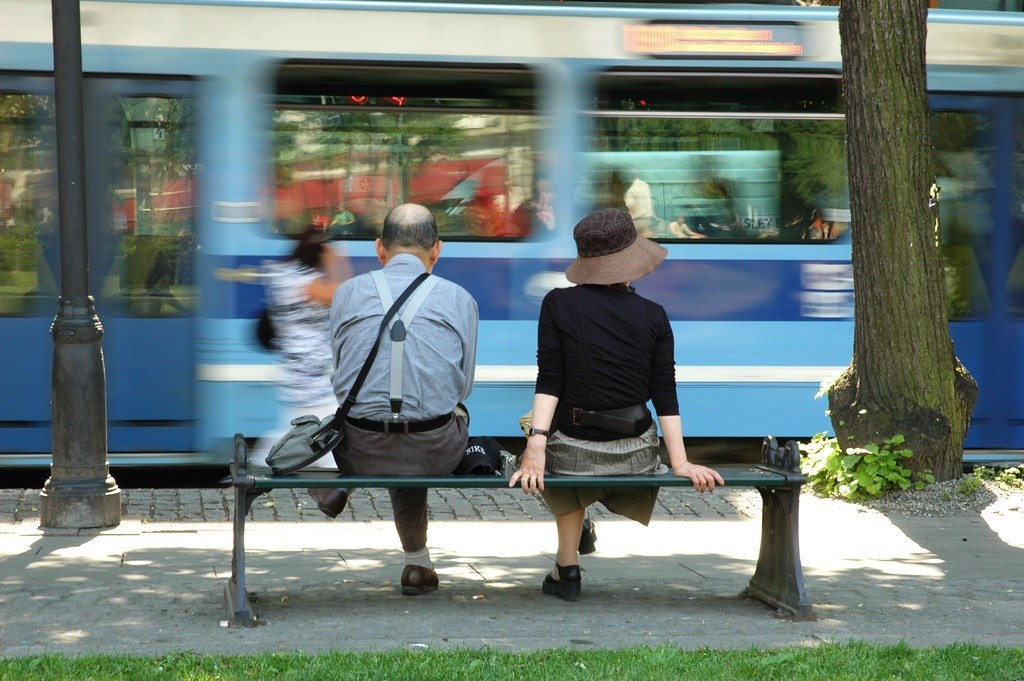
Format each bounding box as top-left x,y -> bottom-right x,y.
300,201 -> 480,593
518,170 -> 711,242
247,226 -> 349,473
508,212 -> 726,603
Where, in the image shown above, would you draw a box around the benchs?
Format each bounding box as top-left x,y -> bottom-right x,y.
219,435 -> 818,630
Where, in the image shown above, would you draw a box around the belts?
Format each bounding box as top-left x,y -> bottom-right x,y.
345,412 -> 453,434
557,404 -> 649,434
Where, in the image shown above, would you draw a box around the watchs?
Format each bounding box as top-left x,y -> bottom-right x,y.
529,427 -> 549,438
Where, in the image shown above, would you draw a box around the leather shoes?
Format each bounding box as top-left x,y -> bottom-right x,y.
308,488 -> 348,519
401,561 -> 439,595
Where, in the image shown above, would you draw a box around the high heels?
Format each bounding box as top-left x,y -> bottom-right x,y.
541,562 -> 581,601
578,511 -> 598,555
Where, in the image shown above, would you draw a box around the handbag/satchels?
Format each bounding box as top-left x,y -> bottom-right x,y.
519,410 -> 534,439
264,414 -> 345,476
256,304 -> 283,353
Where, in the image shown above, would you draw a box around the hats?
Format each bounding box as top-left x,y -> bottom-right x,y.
564,208 -> 669,285
458,436 -> 504,474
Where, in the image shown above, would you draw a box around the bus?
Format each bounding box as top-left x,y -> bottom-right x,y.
0,0 -> 1023,470
